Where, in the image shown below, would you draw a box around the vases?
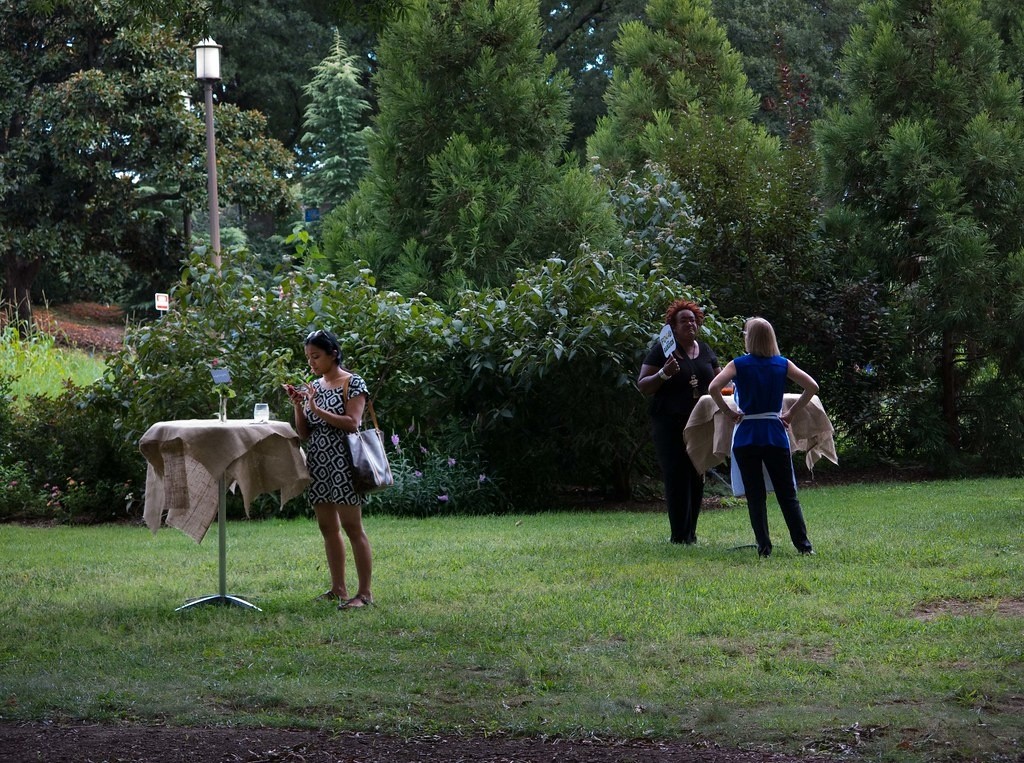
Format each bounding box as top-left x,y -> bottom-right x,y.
218,396 -> 228,420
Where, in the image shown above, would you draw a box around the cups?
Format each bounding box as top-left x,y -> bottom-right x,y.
254,404 -> 269,422
721,380 -> 733,396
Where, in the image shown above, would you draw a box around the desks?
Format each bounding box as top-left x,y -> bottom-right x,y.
138,420 -> 310,613
682,395 -> 839,551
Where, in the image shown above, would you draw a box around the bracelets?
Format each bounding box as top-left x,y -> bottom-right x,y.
659,368 -> 671,379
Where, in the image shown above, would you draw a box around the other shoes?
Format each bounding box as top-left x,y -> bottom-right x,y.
802,549 -> 816,556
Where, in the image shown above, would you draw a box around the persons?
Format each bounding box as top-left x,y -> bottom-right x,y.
637,300 -> 724,545
709,317 -> 820,555
288,330 -> 373,611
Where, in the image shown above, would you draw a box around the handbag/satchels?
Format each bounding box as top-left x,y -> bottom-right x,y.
343,375 -> 394,494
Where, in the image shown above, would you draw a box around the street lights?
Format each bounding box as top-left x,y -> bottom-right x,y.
193,33 -> 224,281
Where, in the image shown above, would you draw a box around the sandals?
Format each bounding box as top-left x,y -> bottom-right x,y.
338,593 -> 374,609
312,589 -> 349,602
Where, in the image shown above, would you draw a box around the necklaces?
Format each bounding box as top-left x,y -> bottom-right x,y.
689,342 -> 699,398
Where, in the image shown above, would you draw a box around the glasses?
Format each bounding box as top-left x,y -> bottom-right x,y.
675,318 -> 698,325
307,330 -> 330,341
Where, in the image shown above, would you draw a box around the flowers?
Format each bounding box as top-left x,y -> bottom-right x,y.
209,358 -> 237,398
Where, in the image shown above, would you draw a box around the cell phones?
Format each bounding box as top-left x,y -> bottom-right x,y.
283,384 -> 303,399
